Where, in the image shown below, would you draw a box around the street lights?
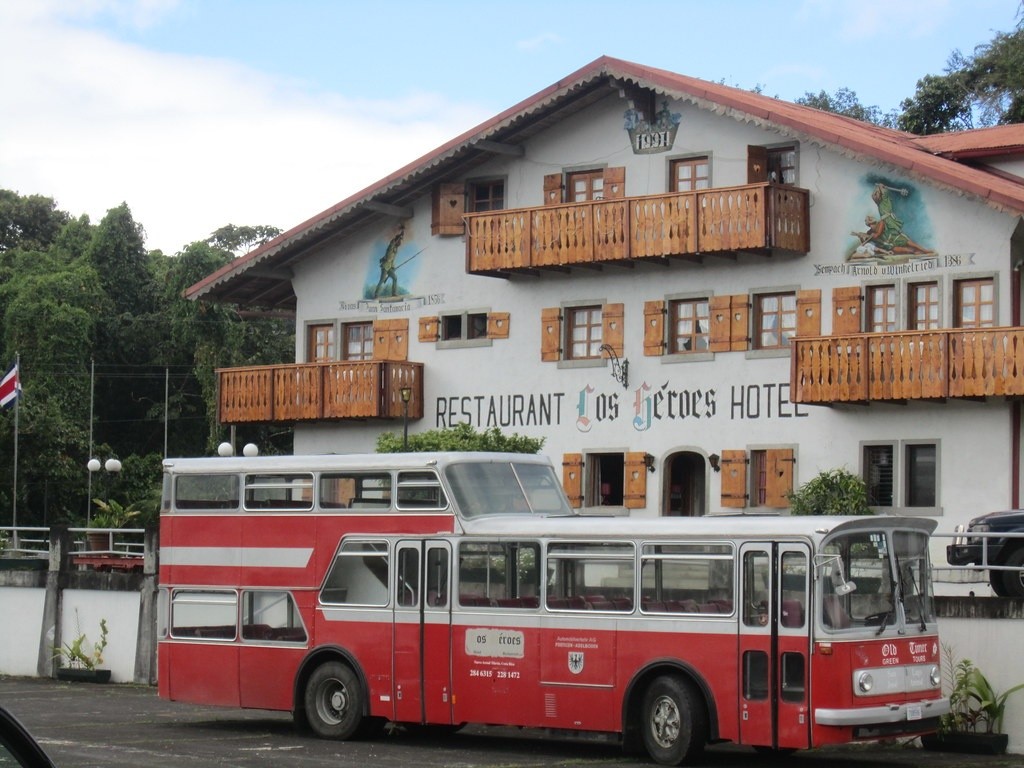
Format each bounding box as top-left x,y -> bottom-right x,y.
88,458 -> 121,515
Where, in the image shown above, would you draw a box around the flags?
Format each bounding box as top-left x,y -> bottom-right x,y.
0,358 -> 22,410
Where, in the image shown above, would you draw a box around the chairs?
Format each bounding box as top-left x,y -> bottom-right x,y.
465,595 -> 802,627
164,623 -> 306,641
176,498 -> 437,511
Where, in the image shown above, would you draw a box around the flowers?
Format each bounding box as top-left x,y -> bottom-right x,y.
491,548 -> 536,583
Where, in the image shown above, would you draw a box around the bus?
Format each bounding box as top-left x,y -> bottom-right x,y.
155,455 -> 950,768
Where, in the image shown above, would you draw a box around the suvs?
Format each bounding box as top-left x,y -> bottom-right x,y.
947,510 -> 1024,597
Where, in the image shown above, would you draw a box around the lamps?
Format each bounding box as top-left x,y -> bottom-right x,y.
643,454 -> 656,473
707,453 -> 721,472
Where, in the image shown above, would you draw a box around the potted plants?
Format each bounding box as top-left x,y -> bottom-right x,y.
920,647 -> 1024,752
47,607 -> 112,682
759,464 -> 881,595
86,498 -> 141,560
